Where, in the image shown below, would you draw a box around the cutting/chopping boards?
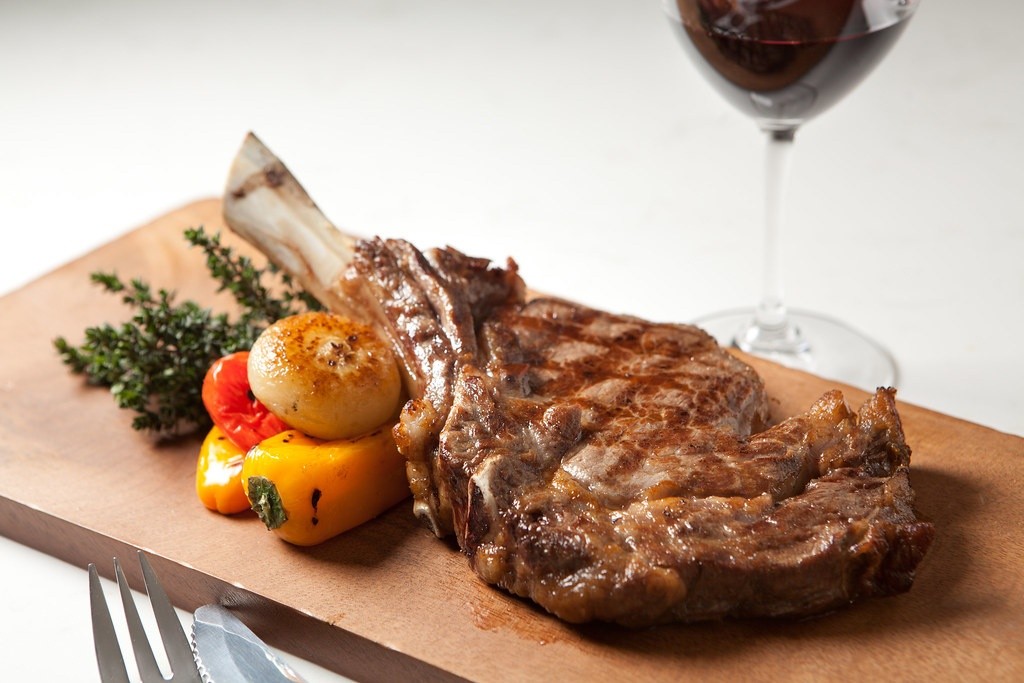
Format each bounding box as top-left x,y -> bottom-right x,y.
1,192 -> 1024,683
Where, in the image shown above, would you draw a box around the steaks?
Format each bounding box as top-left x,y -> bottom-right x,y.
195,131 -> 934,627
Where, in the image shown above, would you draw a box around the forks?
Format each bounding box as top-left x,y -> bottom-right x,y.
84,549 -> 202,683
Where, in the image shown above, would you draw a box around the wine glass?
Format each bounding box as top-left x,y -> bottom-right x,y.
662,1 -> 928,396
